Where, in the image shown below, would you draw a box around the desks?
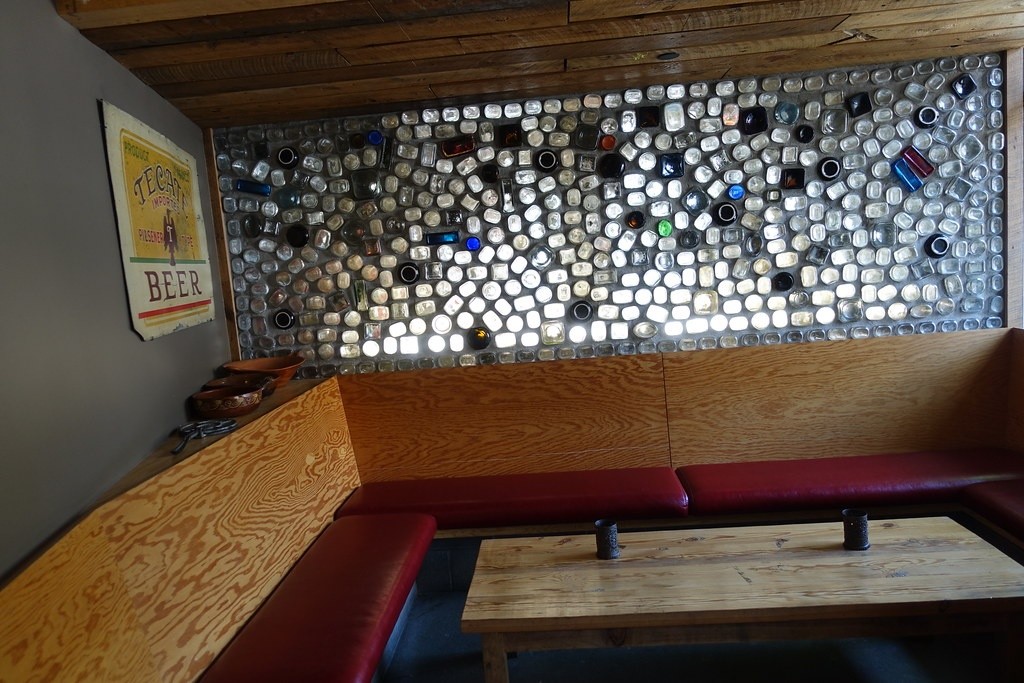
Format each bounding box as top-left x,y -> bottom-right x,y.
457,515 -> 1024,683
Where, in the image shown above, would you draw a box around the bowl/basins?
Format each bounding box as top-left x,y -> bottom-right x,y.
188,354 -> 305,419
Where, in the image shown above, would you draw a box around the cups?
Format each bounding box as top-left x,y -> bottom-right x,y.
842,507 -> 870,550
595,518 -> 620,559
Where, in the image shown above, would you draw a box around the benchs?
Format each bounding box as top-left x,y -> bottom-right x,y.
0,324 -> 1024,683
